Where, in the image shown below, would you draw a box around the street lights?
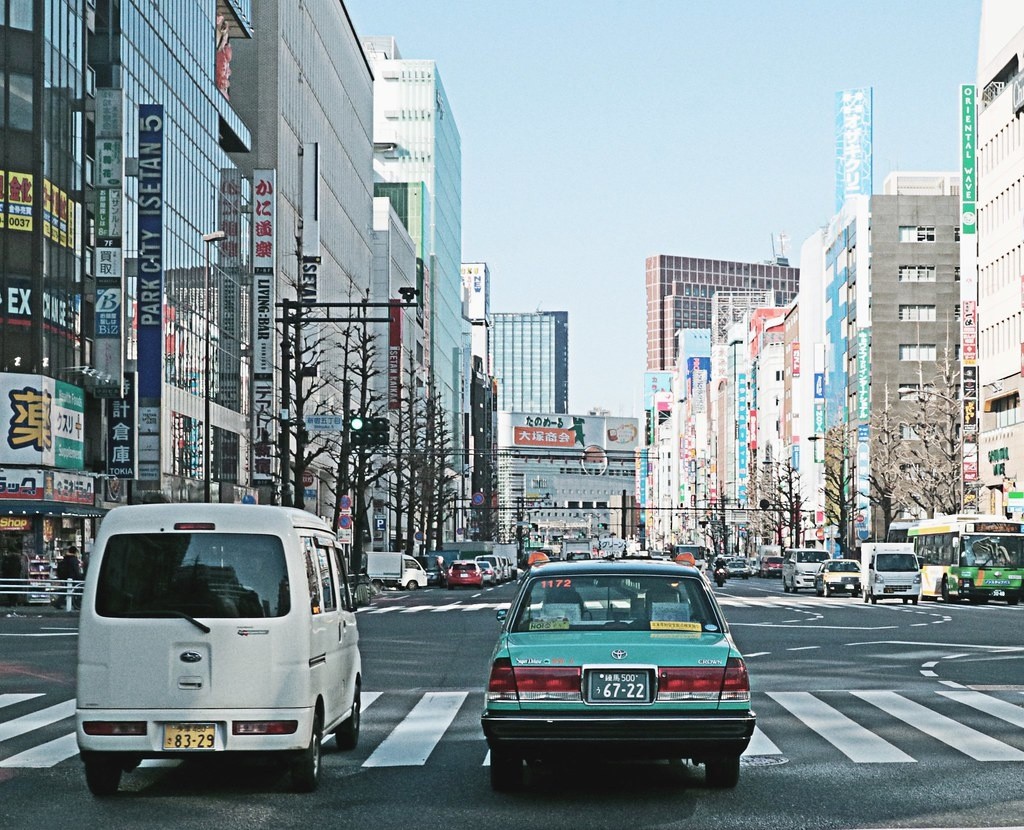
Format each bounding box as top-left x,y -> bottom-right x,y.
202,230 -> 227,501
898,388 -> 963,515
762,456 -> 800,550
808,437 -> 855,559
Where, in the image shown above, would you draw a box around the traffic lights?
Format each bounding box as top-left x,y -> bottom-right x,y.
597,522 -> 607,530
350,418 -> 390,446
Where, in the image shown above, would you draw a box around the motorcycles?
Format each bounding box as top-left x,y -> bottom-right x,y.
711,565 -> 726,587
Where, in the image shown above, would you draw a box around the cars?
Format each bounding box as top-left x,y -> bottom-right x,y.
814,559 -> 861,597
711,554 -> 785,579
481,547 -> 757,792
415,555 -> 513,590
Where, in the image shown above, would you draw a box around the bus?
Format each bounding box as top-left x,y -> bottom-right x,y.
887,513 -> 1024,605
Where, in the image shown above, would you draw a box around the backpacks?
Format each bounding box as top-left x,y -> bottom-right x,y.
57,556 -> 73,579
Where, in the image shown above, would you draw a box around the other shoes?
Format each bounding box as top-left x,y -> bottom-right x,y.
53,603 -> 63,609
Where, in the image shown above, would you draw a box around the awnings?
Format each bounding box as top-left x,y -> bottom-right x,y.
0,500 -> 109,518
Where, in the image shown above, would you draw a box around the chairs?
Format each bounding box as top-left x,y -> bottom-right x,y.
637,589 -> 681,634
551,591 -> 586,621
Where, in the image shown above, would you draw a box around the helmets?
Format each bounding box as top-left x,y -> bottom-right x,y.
717,554 -> 723,562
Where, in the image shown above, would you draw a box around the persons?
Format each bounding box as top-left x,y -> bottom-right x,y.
712,555 -> 728,583
0,548 -> 22,606
52,546 -> 89,609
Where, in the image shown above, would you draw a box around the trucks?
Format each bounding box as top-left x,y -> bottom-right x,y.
441,542 -> 517,580
365,551 -> 430,590
860,544 -> 924,605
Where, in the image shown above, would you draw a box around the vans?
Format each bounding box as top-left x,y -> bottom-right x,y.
673,546 -> 706,576
75,503 -> 372,795
782,549 -> 832,593
